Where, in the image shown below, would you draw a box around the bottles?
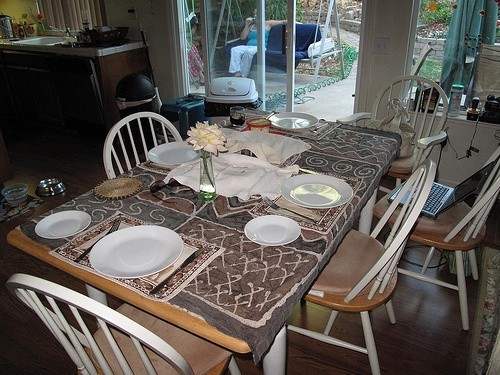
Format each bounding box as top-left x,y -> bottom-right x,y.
413,86 -> 423,112
447,84 -> 464,117
467,98 -> 479,121
422,80 -> 440,113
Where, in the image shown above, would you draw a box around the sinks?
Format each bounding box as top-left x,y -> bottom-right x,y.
11,36 -> 67,47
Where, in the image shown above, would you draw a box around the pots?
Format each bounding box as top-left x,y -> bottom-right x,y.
78,27 -> 129,46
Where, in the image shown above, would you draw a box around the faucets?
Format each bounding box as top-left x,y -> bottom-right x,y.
46,22 -> 72,37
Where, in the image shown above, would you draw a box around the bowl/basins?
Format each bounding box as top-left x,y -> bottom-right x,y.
1,183 -> 29,205
247,117 -> 271,133
35,177 -> 66,197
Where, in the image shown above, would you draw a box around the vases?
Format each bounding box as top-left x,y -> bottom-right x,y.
198,148 -> 216,200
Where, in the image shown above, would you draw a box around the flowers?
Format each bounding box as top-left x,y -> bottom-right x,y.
188,122 -> 228,187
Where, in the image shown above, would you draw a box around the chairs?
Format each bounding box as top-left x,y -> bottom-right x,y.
336,75 -> 449,193
102,112 -> 182,180
375,145 -> 500,330
6,268 -> 240,375
285,157 -> 438,375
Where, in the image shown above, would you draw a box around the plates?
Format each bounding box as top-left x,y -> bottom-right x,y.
35,210 -> 91,239
90,225 -> 184,278
148,142 -> 199,165
269,112 -> 318,129
244,215 -> 301,246
280,174 -> 353,209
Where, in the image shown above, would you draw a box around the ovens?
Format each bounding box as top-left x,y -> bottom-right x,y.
51,54 -> 106,142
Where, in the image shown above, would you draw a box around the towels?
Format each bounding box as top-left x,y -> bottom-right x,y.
160,151 -> 301,203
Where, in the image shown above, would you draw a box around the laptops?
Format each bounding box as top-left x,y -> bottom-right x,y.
388,161 -> 496,221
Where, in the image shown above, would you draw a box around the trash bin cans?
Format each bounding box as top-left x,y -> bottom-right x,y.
115,73 -> 161,152
204,75 -> 262,125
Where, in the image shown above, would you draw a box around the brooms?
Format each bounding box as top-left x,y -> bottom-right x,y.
133,17 -> 166,133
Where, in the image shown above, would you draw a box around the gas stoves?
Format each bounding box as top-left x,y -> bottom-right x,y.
54,39 -> 130,48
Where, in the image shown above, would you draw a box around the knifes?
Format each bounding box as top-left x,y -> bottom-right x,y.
149,247 -> 207,294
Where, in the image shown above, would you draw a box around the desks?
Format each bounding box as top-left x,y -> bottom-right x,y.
7,108 -> 403,375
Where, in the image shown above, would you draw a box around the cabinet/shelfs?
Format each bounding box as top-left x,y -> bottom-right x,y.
405,109 -> 500,187
0,47 -> 156,139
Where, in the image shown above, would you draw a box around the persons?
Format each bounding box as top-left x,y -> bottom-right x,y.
185,17 -> 205,87
228,6 -> 303,78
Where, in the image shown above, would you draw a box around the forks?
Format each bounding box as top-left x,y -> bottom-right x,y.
309,123 -> 328,133
263,196 -> 318,224
74,219 -> 120,263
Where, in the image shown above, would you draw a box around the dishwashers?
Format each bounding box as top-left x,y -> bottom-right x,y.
0,50 -> 64,133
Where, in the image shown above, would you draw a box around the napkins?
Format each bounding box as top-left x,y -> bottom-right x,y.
262,111 -> 341,140
162,130 -> 312,201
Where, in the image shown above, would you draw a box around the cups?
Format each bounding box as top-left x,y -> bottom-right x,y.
230,106 -> 246,127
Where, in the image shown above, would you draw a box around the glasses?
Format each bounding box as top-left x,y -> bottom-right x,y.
150,179 -> 196,204
